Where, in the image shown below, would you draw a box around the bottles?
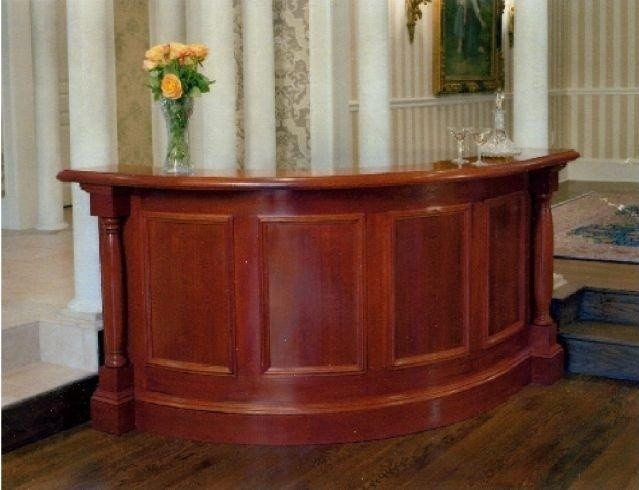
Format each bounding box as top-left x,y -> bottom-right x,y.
480,87 -> 523,158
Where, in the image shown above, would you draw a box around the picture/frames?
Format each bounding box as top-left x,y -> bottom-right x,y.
432,0 -> 506,95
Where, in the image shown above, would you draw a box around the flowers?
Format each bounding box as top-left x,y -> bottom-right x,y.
142,43 -> 213,163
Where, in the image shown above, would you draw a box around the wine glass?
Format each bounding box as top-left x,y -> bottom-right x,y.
447,126 -> 491,165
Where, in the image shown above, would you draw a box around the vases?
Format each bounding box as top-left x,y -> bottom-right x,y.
160,97 -> 193,174
